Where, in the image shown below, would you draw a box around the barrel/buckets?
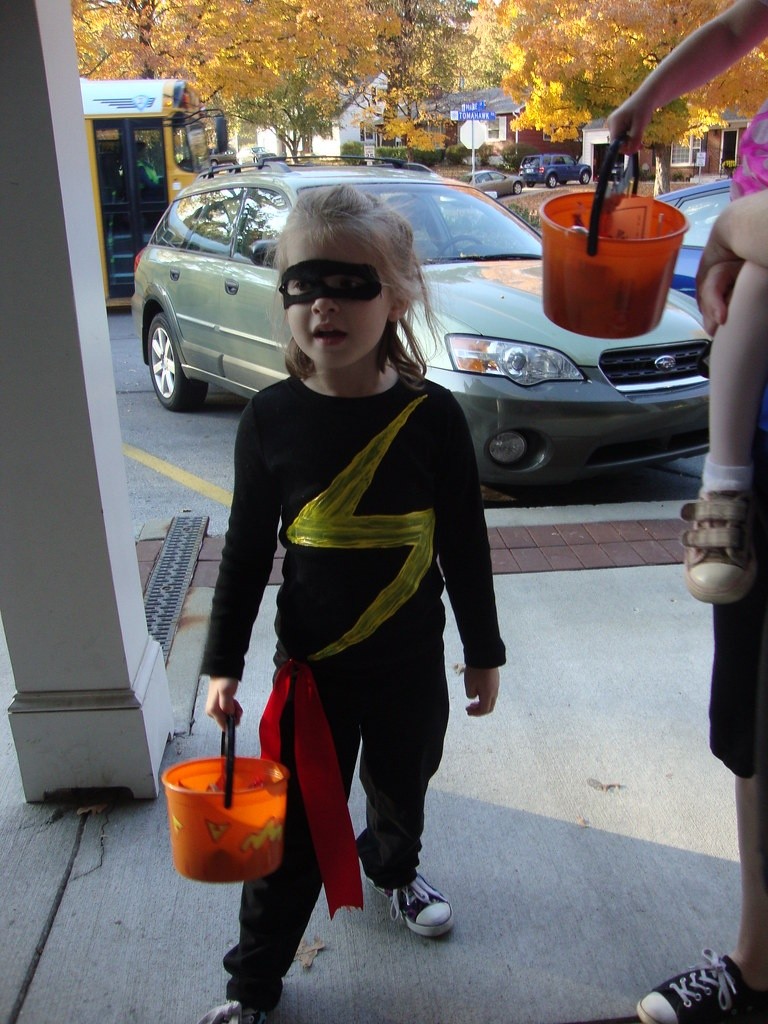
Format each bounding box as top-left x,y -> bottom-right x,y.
161,715 -> 290,883
536,131 -> 692,340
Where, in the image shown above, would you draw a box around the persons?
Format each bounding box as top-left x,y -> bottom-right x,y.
197,185 -> 507,1024
136,140 -> 164,199
605,0 -> 768,1024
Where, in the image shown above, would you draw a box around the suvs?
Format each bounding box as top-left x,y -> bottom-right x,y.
517,152 -> 594,187
207,146 -> 239,166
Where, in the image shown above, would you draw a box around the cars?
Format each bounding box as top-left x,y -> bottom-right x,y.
610,160 -> 625,179
363,164 -> 444,213
130,152 -> 714,501
649,179 -> 733,294
463,166 -> 527,199
237,148 -> 276,165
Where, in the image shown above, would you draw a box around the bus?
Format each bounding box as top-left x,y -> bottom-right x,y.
81,78 -> 230,307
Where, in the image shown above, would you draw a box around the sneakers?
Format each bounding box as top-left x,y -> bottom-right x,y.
637,948 -> 768,1024
365,870 -> 454,937
679,488 -> 755,605
196,1000 -> 266,1024
